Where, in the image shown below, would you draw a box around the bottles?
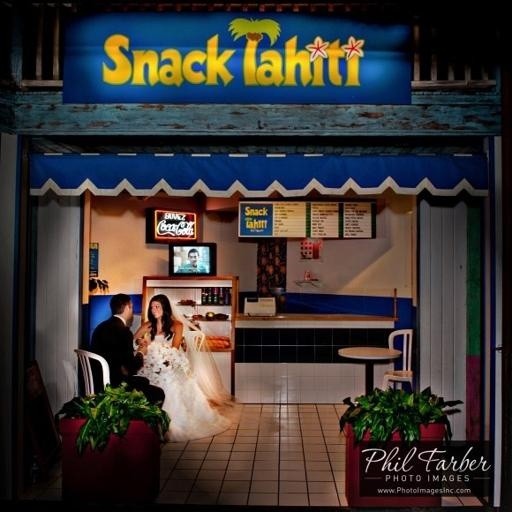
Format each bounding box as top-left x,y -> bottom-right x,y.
207,288 -> 213,306
305,270 -> 311,281
213,288 -> 218,306
201,288 -> 208,305
219,288 -> 225,306
225,289 -> 231,305
313,239 -> 321,259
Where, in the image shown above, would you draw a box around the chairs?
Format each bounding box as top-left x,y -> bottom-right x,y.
187,329 -> 206,350
72,347 -> 111,400
382,328 -> 414,394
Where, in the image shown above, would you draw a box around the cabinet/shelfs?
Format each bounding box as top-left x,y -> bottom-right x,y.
235,313 -> 395,405
142,275 -> 240,401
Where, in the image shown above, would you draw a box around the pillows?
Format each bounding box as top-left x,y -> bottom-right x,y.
337,346 -> 403,397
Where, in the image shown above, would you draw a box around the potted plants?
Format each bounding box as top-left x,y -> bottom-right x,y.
339,384 -> 464,507
52,381 -> 174,503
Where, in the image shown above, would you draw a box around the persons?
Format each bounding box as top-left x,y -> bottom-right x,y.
133,294 -> 231,442
91,293 -> 165,409
176,249 -> 207,273
